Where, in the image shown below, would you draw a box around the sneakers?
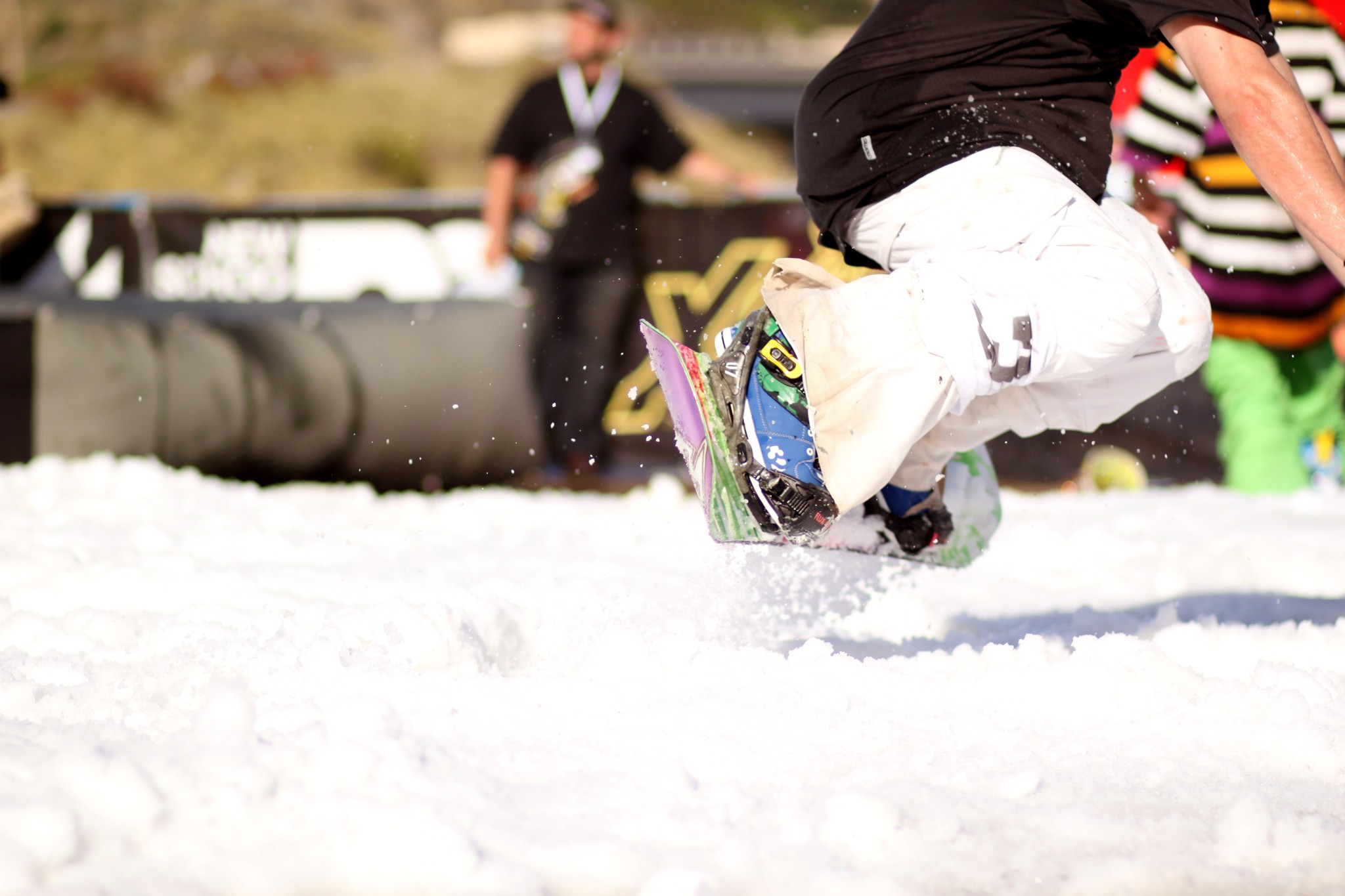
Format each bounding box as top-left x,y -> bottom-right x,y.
877,480 -> 934,520
714,314 -> 827,529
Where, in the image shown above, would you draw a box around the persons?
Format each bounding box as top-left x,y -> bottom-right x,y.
713,0 -> 1345,555
474,0 -> 747,497
1121,0 -> 1345,495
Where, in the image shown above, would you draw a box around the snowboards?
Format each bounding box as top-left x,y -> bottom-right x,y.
639,316 -> 1000,570
